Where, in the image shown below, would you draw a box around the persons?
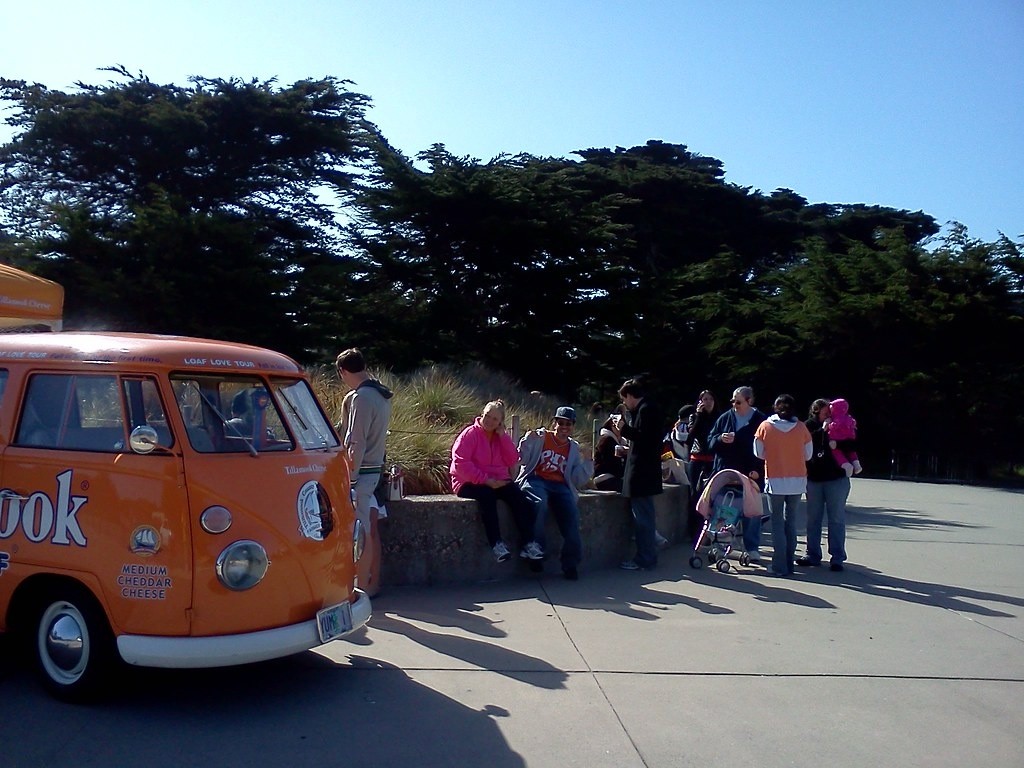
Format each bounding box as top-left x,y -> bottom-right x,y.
223,389 -> 254,438
528,390 -> 545,403
335,348 -> 393,599
449,400 -> 545,564
593,379 -> 862,576
516,407 -> 594,582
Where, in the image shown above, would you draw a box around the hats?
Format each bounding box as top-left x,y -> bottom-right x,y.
554,407 -> 576,420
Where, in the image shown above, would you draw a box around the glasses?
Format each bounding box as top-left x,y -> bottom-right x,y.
557,420 -> 572,426
730,399 -> 743,406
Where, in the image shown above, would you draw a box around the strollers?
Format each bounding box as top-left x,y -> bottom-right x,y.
690,468 -> 764,572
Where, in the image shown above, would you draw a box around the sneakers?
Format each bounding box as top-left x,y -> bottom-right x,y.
493,541 -> 511,563
761,514 -> 770,523
830,563 -> 843,571
748,551 -> 760,561
520,543 -> 544,560
559,558 -> 577,579
655,532 -> 667,545
530,560 -> 545,573
620,558 -> 655,570
766,565 -> 782,576
796,557 -> 822,565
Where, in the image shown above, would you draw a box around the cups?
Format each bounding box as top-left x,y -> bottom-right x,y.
615,445 -> 622,457
728,431 -> 735,442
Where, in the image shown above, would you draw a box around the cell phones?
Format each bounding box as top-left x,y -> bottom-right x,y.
699,400 -> 705,411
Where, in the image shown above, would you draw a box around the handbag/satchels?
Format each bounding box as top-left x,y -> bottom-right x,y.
386,465 -> 403,501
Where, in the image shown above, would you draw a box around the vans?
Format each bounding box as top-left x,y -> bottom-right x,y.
0,331 -> 374,707
